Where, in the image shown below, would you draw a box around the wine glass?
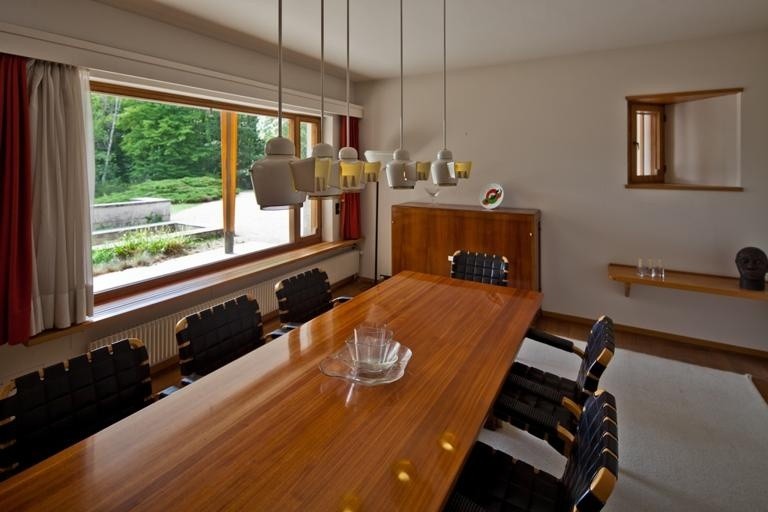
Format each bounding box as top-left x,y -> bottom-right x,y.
425,188 -> 442,208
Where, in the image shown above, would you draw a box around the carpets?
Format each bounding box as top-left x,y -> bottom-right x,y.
464,328 -> 768,512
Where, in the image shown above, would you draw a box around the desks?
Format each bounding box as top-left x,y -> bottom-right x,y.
2,268 -> 544,511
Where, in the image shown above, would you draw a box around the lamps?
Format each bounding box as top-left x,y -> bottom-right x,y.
336,1 -> 382,192
251,1 -> 331,209
385,1 -> 432,191
308,0 -> 364,199
429,2 -> 472,188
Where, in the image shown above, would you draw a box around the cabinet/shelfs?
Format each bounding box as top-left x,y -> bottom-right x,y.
388,201 -> 540,315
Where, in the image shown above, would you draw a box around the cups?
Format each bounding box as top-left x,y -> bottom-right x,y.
634,258 -> 667,280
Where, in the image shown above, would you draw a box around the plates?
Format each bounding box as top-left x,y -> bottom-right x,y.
478,183 -> 505,209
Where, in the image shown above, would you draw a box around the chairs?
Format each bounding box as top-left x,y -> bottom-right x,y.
439,388 -> 621,510
2,337 -> 178,480
449,250 -> 508,286
274,267 -> 353,330
484,316 -> 616,431
175,293 -> 286,386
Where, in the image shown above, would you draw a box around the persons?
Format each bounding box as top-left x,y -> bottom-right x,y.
732,246 -> 768,292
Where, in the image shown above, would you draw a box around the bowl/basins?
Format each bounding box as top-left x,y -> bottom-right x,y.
318,327 -> 414,388
